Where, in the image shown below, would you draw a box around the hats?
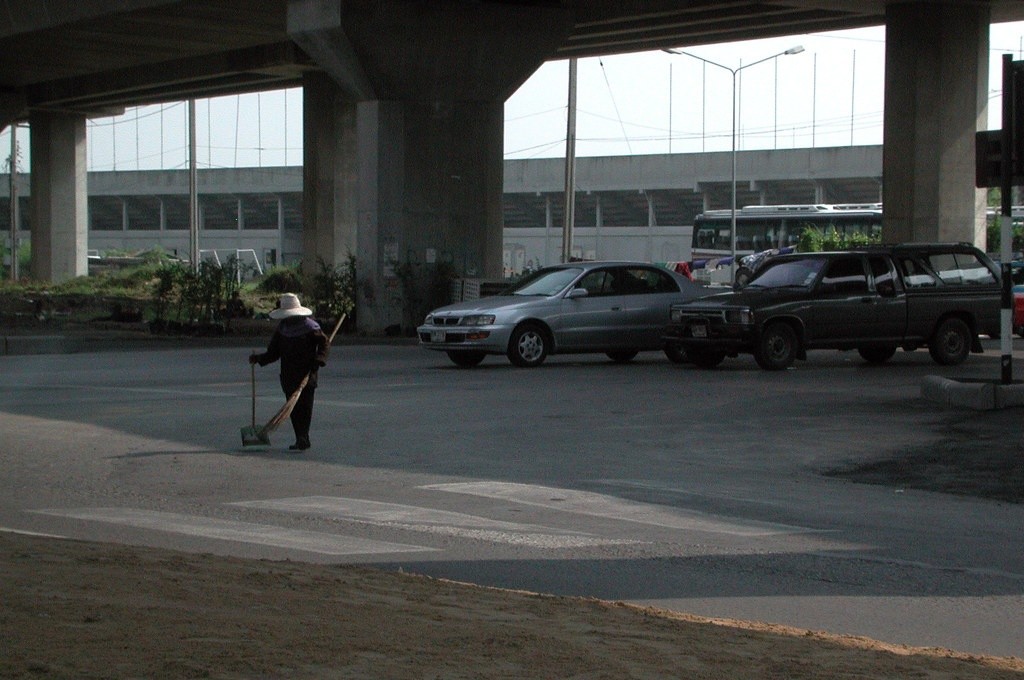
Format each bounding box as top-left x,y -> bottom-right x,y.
269,293 -> 313,320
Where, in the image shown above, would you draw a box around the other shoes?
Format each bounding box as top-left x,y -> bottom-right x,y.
289,440 -> 310,450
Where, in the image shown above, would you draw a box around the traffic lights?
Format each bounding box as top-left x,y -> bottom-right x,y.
974,130 -> 1019,190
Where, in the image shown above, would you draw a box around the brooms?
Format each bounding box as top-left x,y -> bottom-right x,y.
259,313 -> 347,437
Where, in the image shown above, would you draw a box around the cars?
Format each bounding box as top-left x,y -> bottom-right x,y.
414,259 -> 723,367
993,259 -> 1024,342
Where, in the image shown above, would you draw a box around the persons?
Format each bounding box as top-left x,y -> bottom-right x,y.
249,293 -> 331,451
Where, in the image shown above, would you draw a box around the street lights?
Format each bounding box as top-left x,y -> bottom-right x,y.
659,42 -> 806,287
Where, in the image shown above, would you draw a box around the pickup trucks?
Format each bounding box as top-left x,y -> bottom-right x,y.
658,239 -> 1007,370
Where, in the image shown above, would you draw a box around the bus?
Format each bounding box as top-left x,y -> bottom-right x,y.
690,201 -> 882,288
